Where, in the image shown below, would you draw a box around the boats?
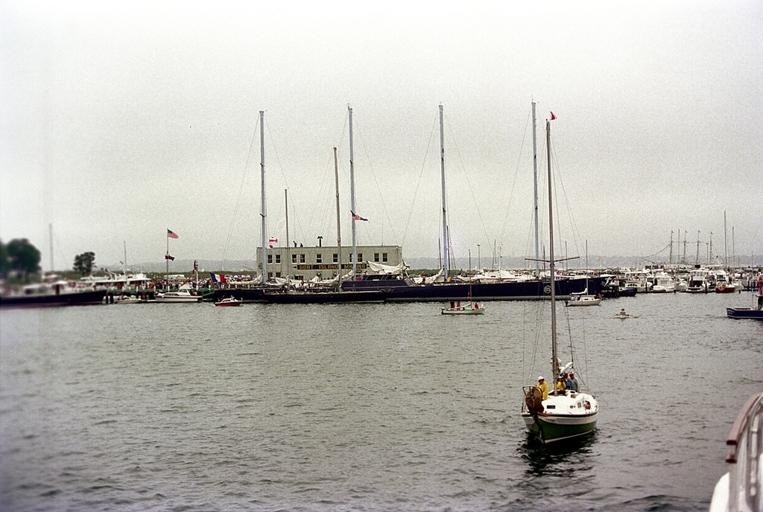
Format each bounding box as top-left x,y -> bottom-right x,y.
0,224 -> 203,304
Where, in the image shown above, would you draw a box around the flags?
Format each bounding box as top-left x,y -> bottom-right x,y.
548,112 -> 557,121
351,210 -> 368,222
166,229 -> 178,239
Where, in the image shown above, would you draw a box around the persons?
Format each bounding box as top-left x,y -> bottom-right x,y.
754,290 -> 763,310
561,372 -> 572,390
567,372 -> 579,393
619,308 -> 626,315
555,377 -> 566,394
534,376 -> 548,401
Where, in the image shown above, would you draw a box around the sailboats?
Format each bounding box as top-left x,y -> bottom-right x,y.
522,118 -> 598,446
